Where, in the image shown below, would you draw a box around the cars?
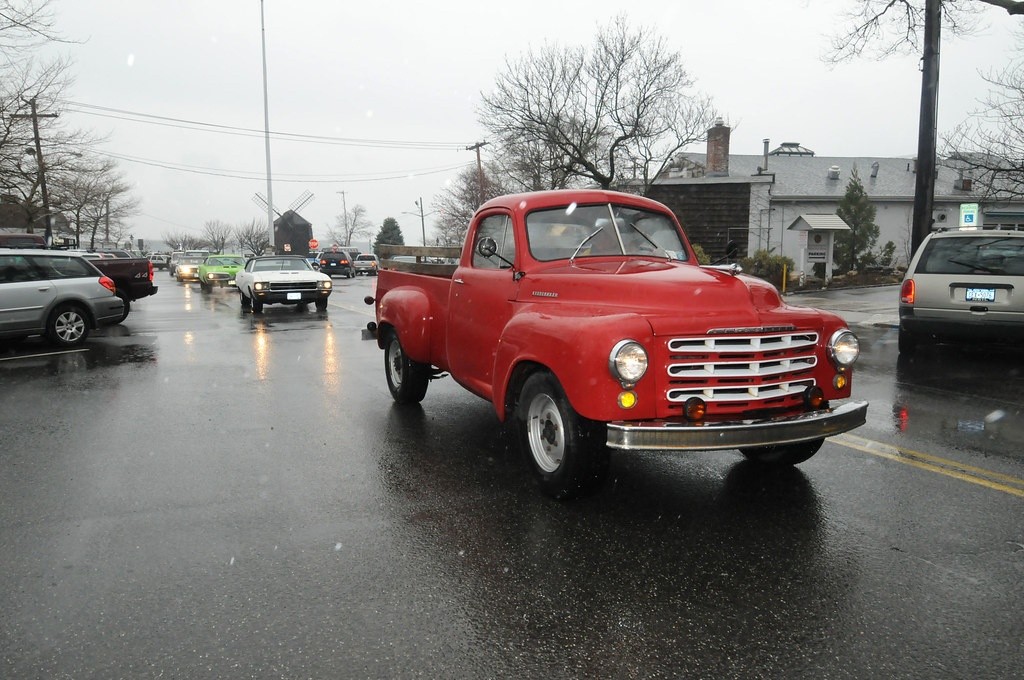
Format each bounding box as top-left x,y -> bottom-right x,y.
306,252 -> 324,271
143,249 -> 246,294
319,252 -> 355,278
234,255 -> 333,312
348,252 -> 361,261
897,230 -> 1024,353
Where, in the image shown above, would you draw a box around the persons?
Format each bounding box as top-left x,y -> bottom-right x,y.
579,217 -> 633,256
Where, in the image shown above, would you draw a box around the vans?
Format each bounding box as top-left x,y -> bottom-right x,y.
0,247 -> 125,348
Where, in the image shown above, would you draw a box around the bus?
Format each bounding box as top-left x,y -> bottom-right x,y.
322,247 -> 358,253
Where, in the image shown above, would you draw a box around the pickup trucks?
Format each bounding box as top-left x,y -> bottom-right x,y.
353,254 -> 379,276
360,189 -> 869,486
0,234 -> 158,303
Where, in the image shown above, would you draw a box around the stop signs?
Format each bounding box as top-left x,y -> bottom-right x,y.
308,238 -> 318,249
332,245 -> 338,251
